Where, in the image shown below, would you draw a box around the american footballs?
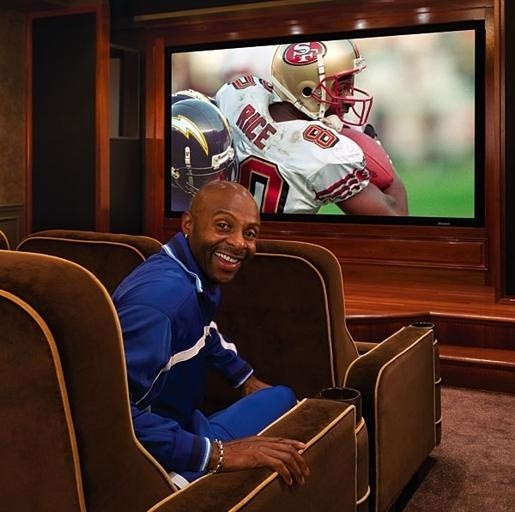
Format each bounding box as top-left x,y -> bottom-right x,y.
340,127 -> 394,191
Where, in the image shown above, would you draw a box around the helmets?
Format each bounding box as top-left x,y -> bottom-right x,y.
271,39 -> 374,127
171,99 -> 241,197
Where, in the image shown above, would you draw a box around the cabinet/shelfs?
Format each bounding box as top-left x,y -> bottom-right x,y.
346,307 -> 515,373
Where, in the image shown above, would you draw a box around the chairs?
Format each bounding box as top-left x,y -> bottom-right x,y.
0,227 -> 442,512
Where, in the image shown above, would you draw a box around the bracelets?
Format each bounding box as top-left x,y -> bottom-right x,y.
211,438 -> 225,474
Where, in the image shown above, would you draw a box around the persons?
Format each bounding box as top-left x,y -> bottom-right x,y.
216,38 -> 408,218
171,88 -> 241,212
113,180 -> 311,487
